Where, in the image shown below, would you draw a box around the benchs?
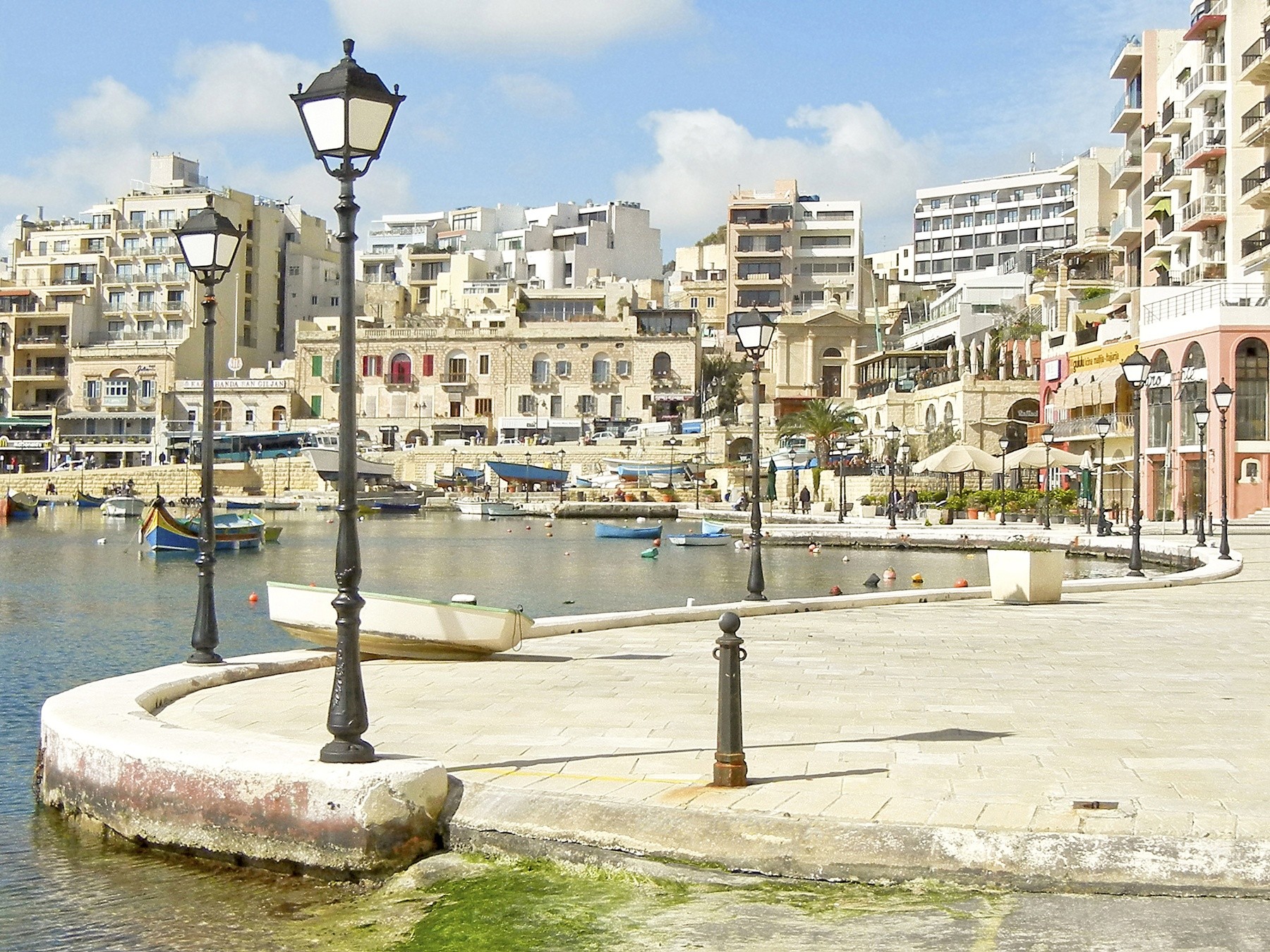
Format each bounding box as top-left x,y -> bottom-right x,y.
579,440 -> 597,445
739,502 -> 749,511
663,440 -> 682,446
781,501 -> 799,513
619,440 -> 637,446
833,502 -> 854,517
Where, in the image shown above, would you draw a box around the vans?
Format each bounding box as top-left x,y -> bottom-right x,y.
624,421 -> 672,437
445,438 -> 470,446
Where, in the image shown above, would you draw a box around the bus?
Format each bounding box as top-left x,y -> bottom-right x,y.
189,430 -> 318,464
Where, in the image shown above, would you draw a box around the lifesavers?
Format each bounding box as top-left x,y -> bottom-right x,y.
196,497 -> 200,504
190,497 -> 195,504
180,497 -> 185,504
185,497 -> 190,505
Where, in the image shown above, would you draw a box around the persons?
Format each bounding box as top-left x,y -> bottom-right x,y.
246,443 -> 263,460
83,454 -> 96,470
733,492 -> 749,512
127,479 -> 134,495
11,457 -> 18,473
45,479 -> 55,495
887,484 -> 917,521
475,429 -> 481,446
483,482 -> 492,502
159,452 -> 165,465
800,486 -> 811,513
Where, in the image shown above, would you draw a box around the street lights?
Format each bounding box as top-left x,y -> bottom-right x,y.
999,346 -> 1236,577
667,421 -> 912,530
171,38 -> 407,764
732,299 -> 778,602
695,376 -> 726,419
530,397 -> 546,444
413,402 -> 427,435
450,448 -> 566,503
273,455 -> 278,497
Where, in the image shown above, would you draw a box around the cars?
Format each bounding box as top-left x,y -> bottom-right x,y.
51,460 -> 86,471
496,438 -> 521,444
591,431 -> 616,439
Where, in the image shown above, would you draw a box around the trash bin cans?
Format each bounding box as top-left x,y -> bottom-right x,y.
640,491 -> 647,502
577,492 -> 583,501
102,487 -> 107,495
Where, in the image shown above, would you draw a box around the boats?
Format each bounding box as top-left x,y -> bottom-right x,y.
265,580 -> 535,661
433,455 -> 732,547
0,467 -> 282,551
758,433 -> 864,470
297,423 -> 423,511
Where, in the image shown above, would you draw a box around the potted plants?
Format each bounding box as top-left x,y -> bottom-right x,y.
860,487 -> 1100,524
987,537 -> 1066,604
456,477 -> 473,493
1156,509 -> 1174,521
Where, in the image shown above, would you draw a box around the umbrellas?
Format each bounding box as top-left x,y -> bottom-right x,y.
910,442 -> 1092,509
766,460 -> 777,517
946,333 -> 1032,379
